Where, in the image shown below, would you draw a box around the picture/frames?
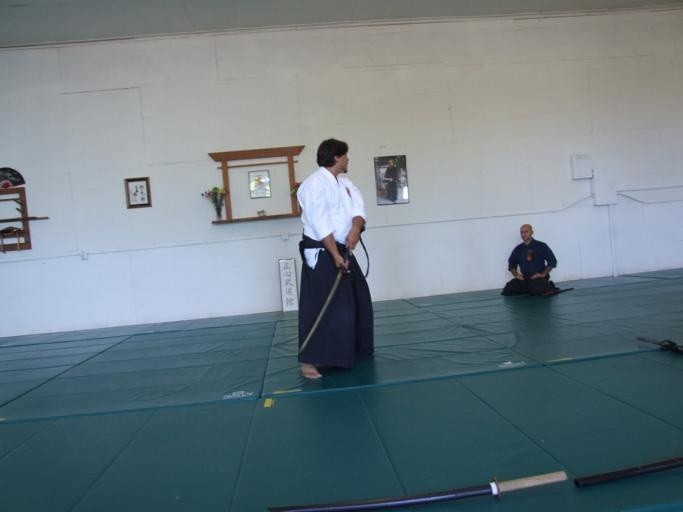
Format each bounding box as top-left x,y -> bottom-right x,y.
248,169 -> 271,198
124,176 -> 152,209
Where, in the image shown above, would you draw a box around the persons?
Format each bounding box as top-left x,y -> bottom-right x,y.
381,159 -> 400,202
295,138 -> 374,379
499,223 -> 560,297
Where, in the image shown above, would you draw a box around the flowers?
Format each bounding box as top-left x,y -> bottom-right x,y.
200,186 -> 227,217
291,180 -> 303,196
